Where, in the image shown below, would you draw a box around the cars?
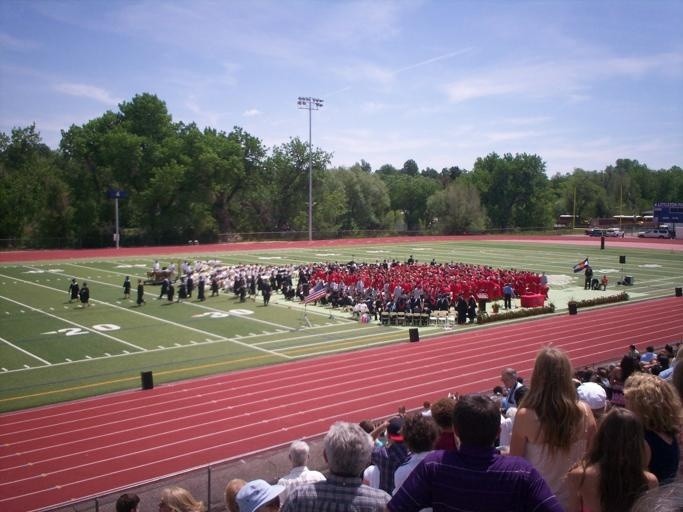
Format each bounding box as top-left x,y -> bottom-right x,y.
584,227 -> 598,236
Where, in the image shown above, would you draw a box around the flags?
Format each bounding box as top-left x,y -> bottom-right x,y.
573,257 -> 589,273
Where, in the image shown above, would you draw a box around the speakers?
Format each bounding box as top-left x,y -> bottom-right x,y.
620,256 -> 625,262
675,288 -> 682,296
142,371 -> 153,390
569,303 -> 577,314
409,329 -> 419,342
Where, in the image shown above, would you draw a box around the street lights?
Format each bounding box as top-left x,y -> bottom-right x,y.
297,95 -> 324,243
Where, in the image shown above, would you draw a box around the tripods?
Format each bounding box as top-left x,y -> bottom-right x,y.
618,263 -> 628,285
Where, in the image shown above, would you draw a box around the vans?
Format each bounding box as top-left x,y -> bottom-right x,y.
591,230 -> 606,237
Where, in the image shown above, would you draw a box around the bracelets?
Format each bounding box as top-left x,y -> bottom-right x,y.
68,291 -> 70,292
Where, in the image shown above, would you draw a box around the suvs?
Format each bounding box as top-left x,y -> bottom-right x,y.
606,228 -> 624,238
638,225 -> 672,240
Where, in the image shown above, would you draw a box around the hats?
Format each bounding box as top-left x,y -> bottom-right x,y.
236,479 -> 285,512
387,420 -> 404,440
576,382 -> 606,409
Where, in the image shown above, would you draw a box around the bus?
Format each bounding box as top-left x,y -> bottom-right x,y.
644,215 -> 655,227
612,215 -> 644,227
557,215 -> 581,225
591,217 -> 618,228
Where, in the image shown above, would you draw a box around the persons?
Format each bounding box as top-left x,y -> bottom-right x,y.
79,282 -> 90,309
123,255 -> 547,324
584,264 -> 608,291
114,340 -> 683,512
68,278 -> 79,303
